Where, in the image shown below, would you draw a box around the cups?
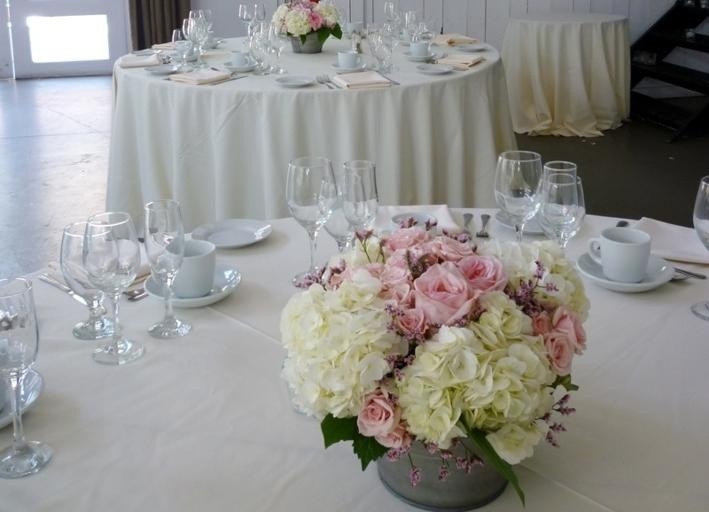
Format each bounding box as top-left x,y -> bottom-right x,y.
410,41 -> 430,57
364,1 -> 436,73
225,49 -> 250,67
348,21 -> 365,40
151,238 -> 217,297
587,224 -> 651,285
334,51 -> 362,74
391,212 -> 439,244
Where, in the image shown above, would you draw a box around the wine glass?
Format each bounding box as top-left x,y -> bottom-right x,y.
490,150 -> 542,242
0,275 -> 55,479
342,161 -> 380,248
61,220 -> 118,342
688,176 -> 709,325
323,174 -> 362,282
141,198 -> 191,343
168,7 -> 215,76
80,210 -> 144,366
282,153 -> 338,291
237,5 -> 285,77
542,163 -> 584,285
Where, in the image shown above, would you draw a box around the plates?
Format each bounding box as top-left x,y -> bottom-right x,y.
403,52 -> 436,63
138,261 -> 243,310
270,74 -> 315,92
456,44 -> 487,54
415,62 -> 455,78
189,215 -> 273,249
0,370 -> 43,430
143,66 -> 177,78
573,249 -> 677,296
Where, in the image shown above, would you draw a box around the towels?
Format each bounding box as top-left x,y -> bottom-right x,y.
437,53 -> 485,70
434,34 -> 478,48
169,66 -> 230,85
119,52 -> 162,69
152,43 -> 178,50
332,70 -> 393,90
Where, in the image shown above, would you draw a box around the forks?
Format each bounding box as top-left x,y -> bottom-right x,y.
322,75 -> 342,91
476,214 -> 492,239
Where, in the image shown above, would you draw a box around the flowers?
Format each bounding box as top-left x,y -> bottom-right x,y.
269,0 -> 343,43
278,216 -> 590,486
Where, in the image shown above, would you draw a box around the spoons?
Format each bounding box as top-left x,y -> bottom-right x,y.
317,76 -> 335,92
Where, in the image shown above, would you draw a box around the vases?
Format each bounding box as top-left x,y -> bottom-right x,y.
286,28 -> 329,54
376,435 -> 513,512
